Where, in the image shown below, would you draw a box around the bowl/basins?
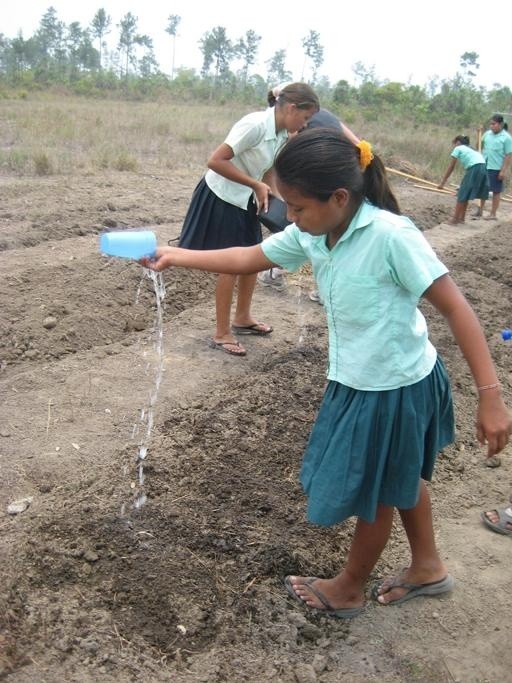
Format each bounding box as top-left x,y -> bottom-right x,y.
257,192 -> 290,232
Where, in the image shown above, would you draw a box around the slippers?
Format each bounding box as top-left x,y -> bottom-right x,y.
232,323 -> 273,334
284,574 -> 361,618
388,567 -> 453,605
210,341 -> 246,356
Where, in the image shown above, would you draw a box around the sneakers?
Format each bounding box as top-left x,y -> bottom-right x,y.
310,290 -> 319,301
257,269 -> 286,291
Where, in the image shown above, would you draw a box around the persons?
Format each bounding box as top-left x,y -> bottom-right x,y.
139,125 -> 512,609
177,81 -> 320,353
472,113 -> 511,218
437,133 -> 486,224
257,80 -> 361,290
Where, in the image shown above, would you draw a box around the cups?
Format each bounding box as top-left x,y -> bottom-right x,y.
98,228 -> 159,261
503,327 -> 511,342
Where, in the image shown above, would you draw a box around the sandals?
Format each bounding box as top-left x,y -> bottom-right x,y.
481,506 -> 511,536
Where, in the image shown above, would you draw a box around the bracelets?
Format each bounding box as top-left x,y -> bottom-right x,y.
476,381 -> 504,390
